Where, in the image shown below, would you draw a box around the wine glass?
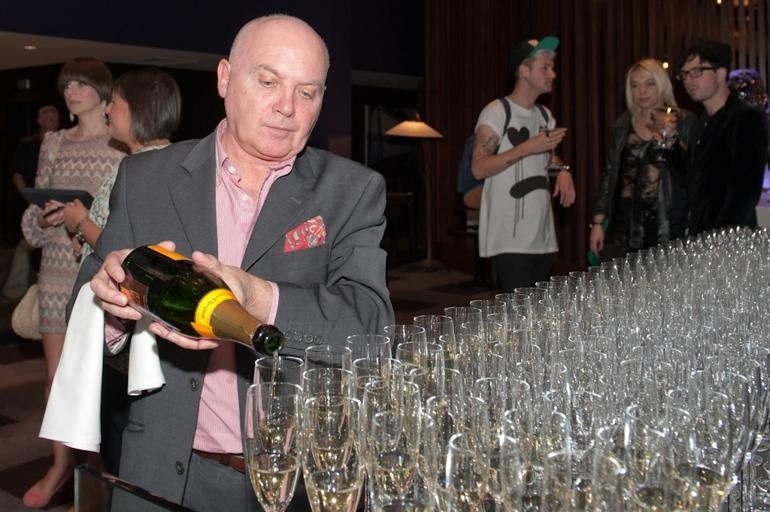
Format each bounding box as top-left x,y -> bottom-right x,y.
242,224 -> 770,512
545,129 -> 564,170
652,108 -> 679,150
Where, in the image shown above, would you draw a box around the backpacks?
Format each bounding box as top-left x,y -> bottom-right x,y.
456,98 -> 548,208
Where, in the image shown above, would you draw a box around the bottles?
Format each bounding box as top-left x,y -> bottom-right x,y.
117,245 -> 286,357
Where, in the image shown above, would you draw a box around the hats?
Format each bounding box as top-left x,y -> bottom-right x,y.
512,36 -> 560,72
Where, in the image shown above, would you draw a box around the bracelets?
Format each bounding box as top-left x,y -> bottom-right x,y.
76,217 -> 94,243
562,164 -> 573,172
592,222 -> 600,226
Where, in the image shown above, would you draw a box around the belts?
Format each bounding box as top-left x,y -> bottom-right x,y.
214,454 -> 246,473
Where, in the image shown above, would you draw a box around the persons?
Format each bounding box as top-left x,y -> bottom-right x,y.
0,105 -> 61,304
62,13 -> 397,512
59,64 -> 181,494
470,35 -> 575,292
589,58 -> 697,263
681,33 -> 769,234
19,55 -> 116,509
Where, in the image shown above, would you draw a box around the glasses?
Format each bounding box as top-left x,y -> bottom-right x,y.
674,67 -> 718,80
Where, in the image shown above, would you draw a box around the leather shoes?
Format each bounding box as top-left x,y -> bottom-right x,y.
23,456 -> 75,508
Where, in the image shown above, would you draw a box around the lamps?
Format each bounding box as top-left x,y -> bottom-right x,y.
382,110 -> 444,273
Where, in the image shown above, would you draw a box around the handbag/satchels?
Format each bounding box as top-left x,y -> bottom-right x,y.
20,187 -> 94,210
12,285 -> 42,340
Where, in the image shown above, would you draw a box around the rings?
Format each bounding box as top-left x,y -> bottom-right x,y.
546,132 -> 551,137
